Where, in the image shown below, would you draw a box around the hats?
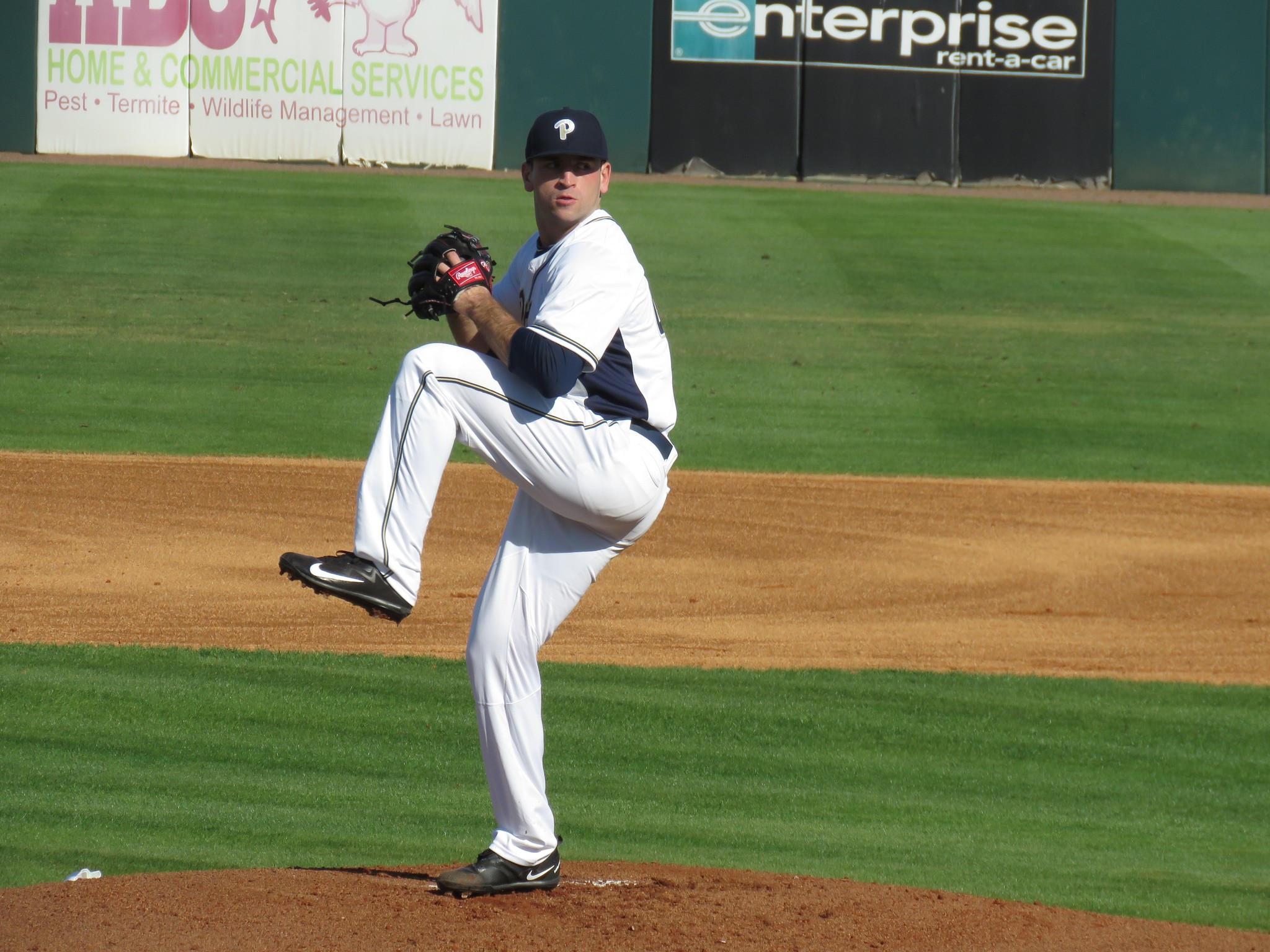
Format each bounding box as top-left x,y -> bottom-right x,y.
524,107 -> 608,162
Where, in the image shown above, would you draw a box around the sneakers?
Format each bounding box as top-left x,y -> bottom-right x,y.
280,549 -> 412,625
437,834 -> 563,898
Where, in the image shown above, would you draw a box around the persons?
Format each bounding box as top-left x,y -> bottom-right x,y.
280,107 -> 678,899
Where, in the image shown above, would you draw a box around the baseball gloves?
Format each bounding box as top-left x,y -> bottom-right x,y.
409,228 -> 495,321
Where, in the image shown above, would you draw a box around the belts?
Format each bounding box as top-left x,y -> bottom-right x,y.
607,414 -> 672,459
